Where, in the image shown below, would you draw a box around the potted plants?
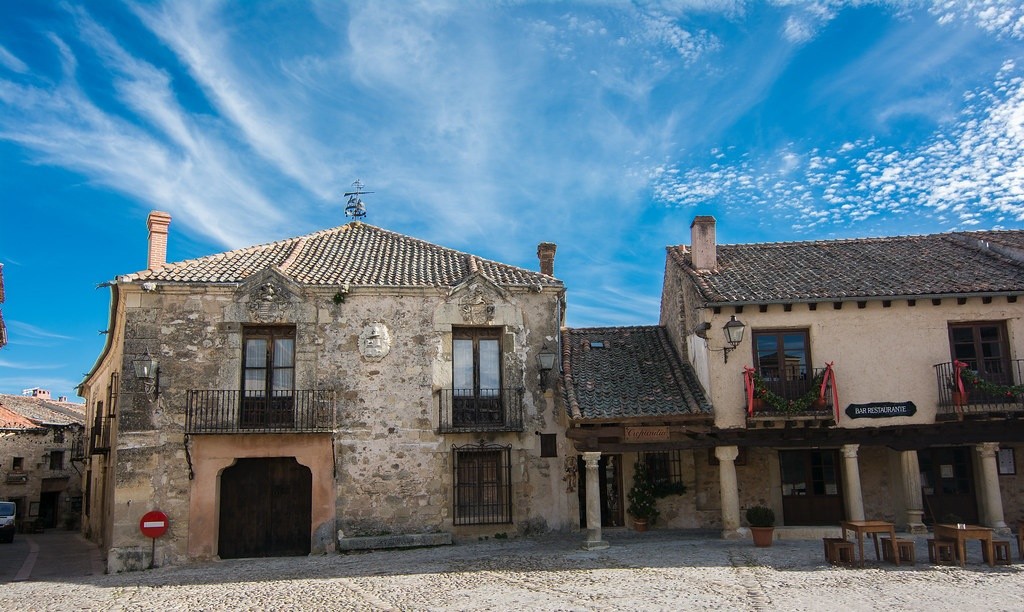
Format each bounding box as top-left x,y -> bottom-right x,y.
746,369 -> 832,412
746,506 -> 775,548
627,464 -> 660,532
947,372 -> 970,406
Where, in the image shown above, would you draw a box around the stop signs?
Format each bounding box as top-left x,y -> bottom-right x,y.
141,511 -> 168,538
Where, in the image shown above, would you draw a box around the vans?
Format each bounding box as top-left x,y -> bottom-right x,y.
0,501 -> 17,543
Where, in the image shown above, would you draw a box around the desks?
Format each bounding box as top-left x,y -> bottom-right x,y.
840,521 -> 900,569
931,523 -> 994,568
1009,520 -> 1024,561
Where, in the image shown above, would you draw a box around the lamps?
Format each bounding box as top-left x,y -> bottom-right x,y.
132,346 -> 162,403
722,314 -> 745,365
535,342 -> 556,393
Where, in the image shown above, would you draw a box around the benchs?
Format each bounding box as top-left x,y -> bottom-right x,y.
979,537 -> 1011,565
880,538 -> 916,566
926,539 -> 956,566
823,538 -> 855,566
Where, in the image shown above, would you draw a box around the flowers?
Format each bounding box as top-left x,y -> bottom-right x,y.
952,361 -> 1024,398
